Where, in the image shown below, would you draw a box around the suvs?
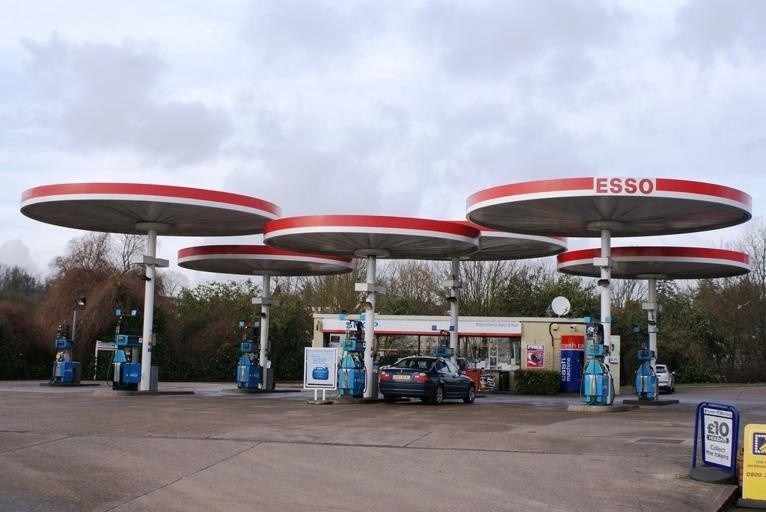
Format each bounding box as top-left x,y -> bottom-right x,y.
655,364 -> 676,394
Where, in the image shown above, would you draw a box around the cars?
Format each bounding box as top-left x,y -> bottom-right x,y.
378,355 -> 476,404
